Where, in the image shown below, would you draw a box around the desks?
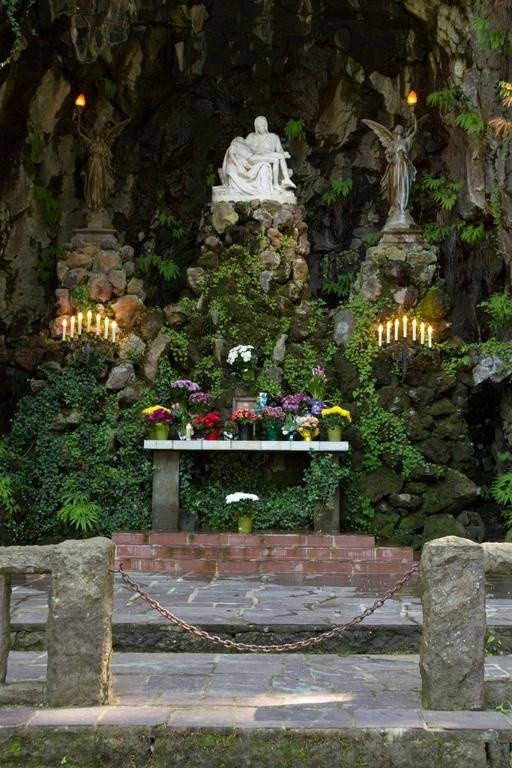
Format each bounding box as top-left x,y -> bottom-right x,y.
144,439 -> 349,534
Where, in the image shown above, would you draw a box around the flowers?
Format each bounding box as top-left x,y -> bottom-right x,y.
225,488 -> 260,517
139,345 -> 354,429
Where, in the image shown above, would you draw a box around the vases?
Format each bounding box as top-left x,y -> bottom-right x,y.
149,429 -> 168,440
175,428 -> 190,440
236,516 -> 253,532
328,429 -> 342,441
265,429 -> 279,441
300,429 -> 313,441
240,428 -> 252,440
206,428 -> 221,440
285,429 -> 298,441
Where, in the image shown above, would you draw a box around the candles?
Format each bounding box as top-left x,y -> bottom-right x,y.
61,310 -> 117,344
375,316 -> 434,349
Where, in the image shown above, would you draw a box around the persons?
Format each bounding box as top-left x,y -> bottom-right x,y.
244,115 -> 294,193
228,137 -> 296,193
77,123 -> 115,211
384,111 -> 417,216
